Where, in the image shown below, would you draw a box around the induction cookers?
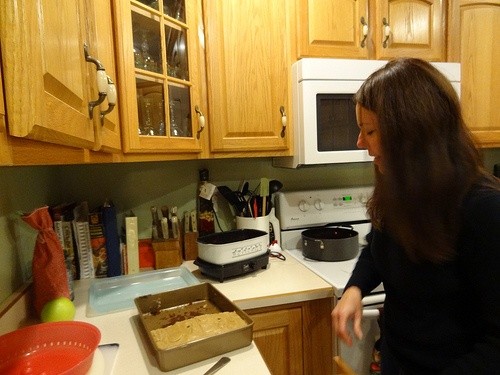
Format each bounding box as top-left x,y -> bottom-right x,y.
275,186 -> 386,298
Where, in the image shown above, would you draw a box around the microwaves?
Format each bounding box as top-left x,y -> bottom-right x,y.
272,56 -> 463,170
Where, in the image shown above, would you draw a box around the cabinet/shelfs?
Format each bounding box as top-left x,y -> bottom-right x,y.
447,0 -> 500,150
0,0 -> 123,168
243,297 -> 337,375
109,0 -> 207,162
295,0 -> 447,62
202,0 -> 299,160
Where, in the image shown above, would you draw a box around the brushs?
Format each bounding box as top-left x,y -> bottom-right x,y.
260,177 -> 270,217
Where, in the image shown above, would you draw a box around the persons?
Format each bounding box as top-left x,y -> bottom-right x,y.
331,58 -> 500,375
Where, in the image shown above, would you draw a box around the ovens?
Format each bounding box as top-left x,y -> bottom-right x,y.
337,293 -> 386,375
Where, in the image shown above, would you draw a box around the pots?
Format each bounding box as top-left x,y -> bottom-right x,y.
192,229 -> 272,282
301,222 -> 360,260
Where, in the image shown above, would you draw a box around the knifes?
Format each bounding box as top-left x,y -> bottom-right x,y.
150,206 -> 180,239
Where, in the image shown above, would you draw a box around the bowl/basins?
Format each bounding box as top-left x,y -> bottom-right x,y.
134,282 -> 254,372
0,321 -> 102,375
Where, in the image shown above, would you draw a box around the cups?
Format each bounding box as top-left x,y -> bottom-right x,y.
236,207 -> 280,248
139,96 -> 187,138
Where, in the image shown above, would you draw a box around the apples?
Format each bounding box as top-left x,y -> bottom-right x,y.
41,297 -> 75,322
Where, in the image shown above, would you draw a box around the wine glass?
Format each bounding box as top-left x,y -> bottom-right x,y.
131,23 -> 159,72
